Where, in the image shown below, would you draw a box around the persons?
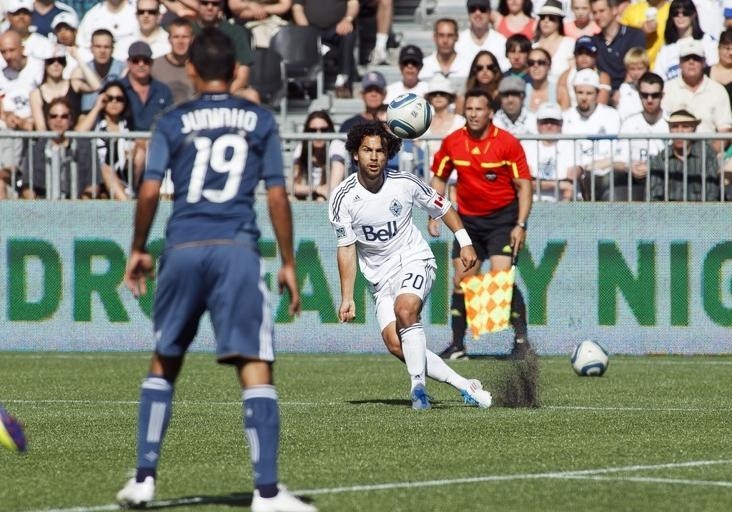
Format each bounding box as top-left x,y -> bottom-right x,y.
427,90 -> 535,362
2,1 -> 732,203
116,29 -> 321,512
0,405 -> 28,455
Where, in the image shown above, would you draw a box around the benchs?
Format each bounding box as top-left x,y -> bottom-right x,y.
250,0 -> 434,148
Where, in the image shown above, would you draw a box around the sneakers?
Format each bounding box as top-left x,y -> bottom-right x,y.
251,483 -> 317,512
438,345 -> 469,362
464,378 -> 492,408
410,384 -> 432,410
115,477 -> 154,504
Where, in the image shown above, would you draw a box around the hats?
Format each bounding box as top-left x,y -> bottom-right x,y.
499,74 -> 528,94
575,35 -> 600,57
574,70 -> 611,93
535,0 -> 566,18
398,47 -> 422,70
663,103 -> 701,124
679,42 -> 707,59
6,1 -> 35,16
537,101 -> 565,123
361,71 -> 387,92
427,76 -> 454,98
639,90 -> 662,98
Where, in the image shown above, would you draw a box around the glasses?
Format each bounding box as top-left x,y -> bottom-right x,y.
307,126 -> 331,132
671,7 -> 693,18
474,63 -> 499,73
48,9 -> 158,120
527,58 -> 549,68
468,3 -> 487,13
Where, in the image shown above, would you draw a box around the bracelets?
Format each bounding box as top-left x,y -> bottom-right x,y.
454,228 -> 473,250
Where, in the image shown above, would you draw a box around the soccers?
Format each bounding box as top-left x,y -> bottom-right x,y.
570,340 -> 608,376
388,94 -> 431,139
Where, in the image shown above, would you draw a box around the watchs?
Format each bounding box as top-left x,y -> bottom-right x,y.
516,221 -> 527,229
328,121 -> 495,410
